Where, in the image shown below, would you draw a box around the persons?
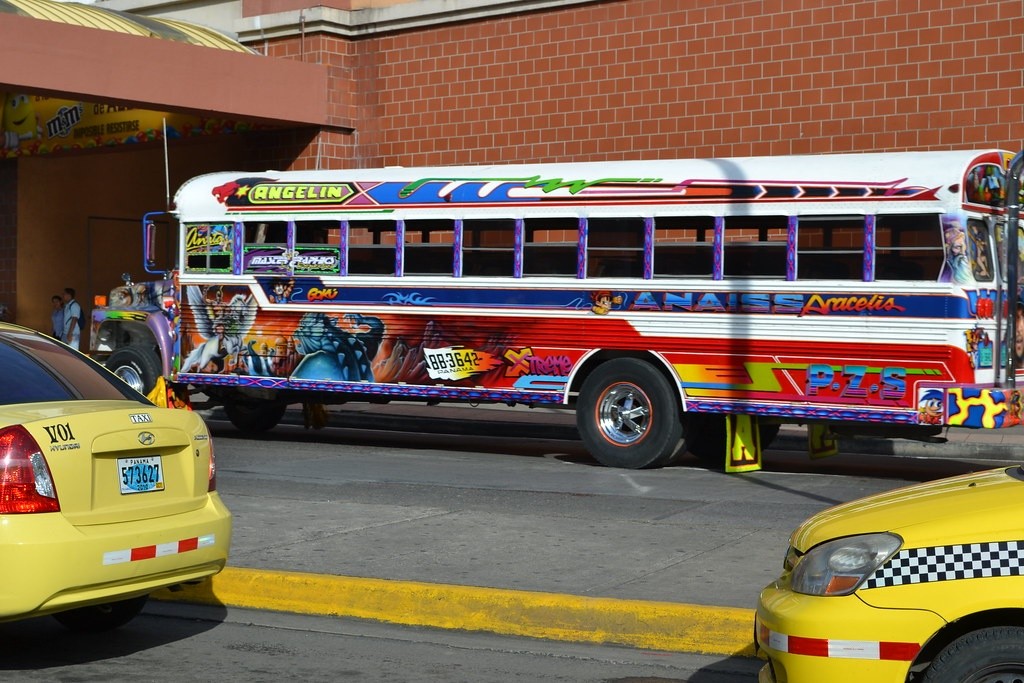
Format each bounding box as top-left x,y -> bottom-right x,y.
1014,303 -> 1024,370
59,287 -> 81,351
50,295 -> 66,341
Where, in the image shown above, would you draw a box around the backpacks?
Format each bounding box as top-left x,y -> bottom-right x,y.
66,300 -> 85,330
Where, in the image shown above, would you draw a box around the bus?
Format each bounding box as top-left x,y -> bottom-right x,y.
90,149 -> 1024,470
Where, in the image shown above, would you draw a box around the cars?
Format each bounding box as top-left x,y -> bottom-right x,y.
751,460 -> 1024,682
1,321 -> 234,631
